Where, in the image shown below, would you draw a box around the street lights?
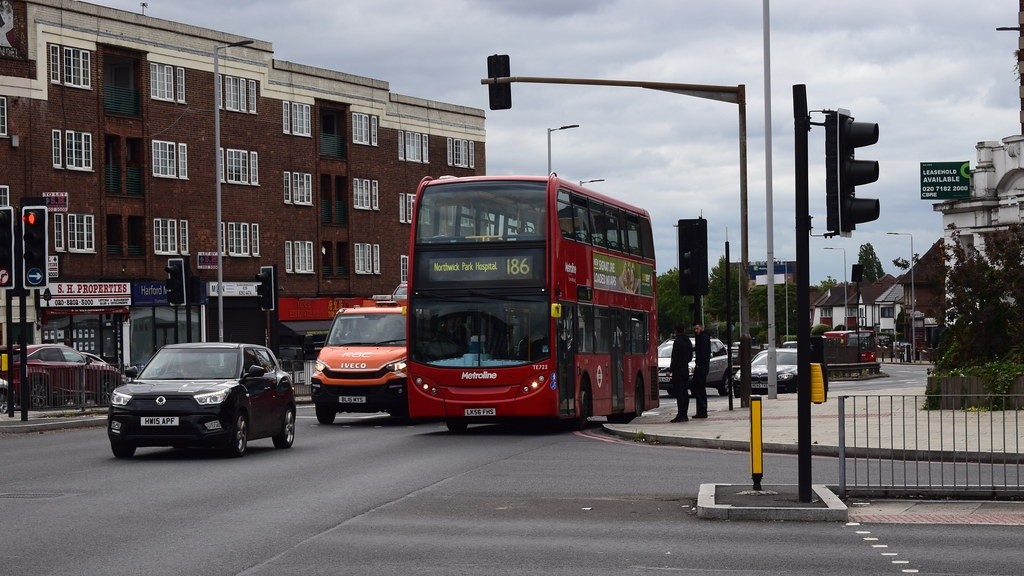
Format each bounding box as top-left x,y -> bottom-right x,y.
548,124 -> 580,177
887,232 -> 916,364
213,39 -> 256,370
774,257 -> 788,342
823,247 -> 847,330
580,179 -> 605,186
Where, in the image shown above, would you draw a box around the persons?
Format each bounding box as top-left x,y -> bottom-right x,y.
667,324 -> 694,422
689,323 -> 711,419
911,345 -> 920,361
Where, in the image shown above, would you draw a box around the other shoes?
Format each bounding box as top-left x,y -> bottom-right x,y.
670,414 -> 689,422
692,414 -> 708,419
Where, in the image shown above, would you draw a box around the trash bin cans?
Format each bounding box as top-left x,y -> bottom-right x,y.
912,347 -> 920,360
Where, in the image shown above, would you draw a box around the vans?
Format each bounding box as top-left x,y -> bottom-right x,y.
311,301 -> 407,423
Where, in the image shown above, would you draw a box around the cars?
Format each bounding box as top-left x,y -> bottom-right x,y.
107,341 -> 297,457
80,351 -> 106,363
0,378 -> 16,414
0,343 -> 126,409
723,340 -> 798,364
734,348 -> 799,398
658,336 -> 733,395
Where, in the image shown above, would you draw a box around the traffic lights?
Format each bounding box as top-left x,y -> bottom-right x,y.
0,207 -> 17,289
21,206 -> 49,290
677,219 -> 709,296
255,266 -> 275,311
164,258 -> 186,306
826,108 -> 882,237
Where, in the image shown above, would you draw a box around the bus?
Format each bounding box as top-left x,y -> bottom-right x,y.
823,330 -> 877,363
402,173 -> 661,432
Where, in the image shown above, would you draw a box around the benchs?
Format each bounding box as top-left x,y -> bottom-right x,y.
517,225 -> 641,255
